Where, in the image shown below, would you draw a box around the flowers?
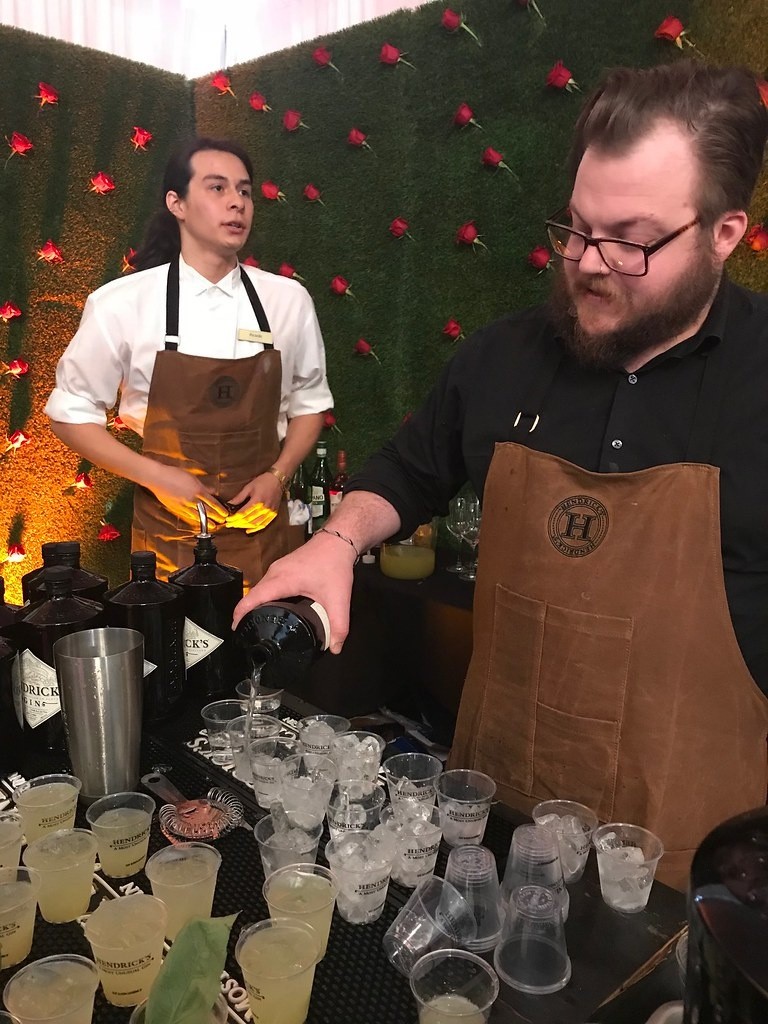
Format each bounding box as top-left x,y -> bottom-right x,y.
0,0 -> 768,562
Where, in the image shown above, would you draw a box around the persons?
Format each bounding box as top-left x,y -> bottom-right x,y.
230,52 -> 768,894
43,130 -> 335,599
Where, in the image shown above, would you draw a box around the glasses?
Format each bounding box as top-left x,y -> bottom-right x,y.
543,203 -> 703,277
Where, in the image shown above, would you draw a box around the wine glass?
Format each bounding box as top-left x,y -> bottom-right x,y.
448,492 -> 482,584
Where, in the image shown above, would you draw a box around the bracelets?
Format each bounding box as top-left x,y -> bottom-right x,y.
313,529 -> 360,567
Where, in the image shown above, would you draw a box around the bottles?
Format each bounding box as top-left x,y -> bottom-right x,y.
378,516 -> 438,579
284,441 -> 349,543
0,536 -> 330,797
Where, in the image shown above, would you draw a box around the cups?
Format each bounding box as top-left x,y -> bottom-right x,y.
683,803 -> 768,1024
0,680 -> 665,1024
51,627 -> 153,808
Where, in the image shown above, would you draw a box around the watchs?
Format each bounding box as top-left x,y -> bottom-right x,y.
267,467 -> 291,493
